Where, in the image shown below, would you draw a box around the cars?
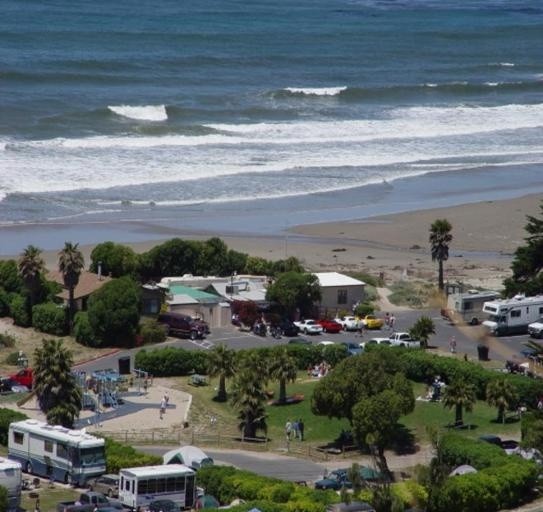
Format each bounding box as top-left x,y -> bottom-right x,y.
282,315 -> 421,355
315,465 -> 383,490
326,501 -> 376,512
480,434 -> 543,464
57,474 -> 130,512
0,369 -> 34,393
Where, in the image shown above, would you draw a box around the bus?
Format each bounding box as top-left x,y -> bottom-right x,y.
448,288 -> 543,338
0,419 -> 107,508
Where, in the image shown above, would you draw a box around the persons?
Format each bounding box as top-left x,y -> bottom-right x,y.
159,392 -> 169,420
449,336 -> 456,353
385,312 -> 396,333
307,360 -> 331,378
285,419 -> 292,441
425,378 -> 441,401
298,418 -> 304,441
292,420 -> 298,438
355,318 -> 365,338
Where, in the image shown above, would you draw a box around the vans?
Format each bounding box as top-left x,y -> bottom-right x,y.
118,464 -> 205,512
156,312 -> 211,339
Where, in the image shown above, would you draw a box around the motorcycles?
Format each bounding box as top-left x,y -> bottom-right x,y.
254,322 -> 282,339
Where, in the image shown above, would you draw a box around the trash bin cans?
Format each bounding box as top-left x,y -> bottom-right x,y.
135,335 -> 143,346
119,356 -> 129,373
472,318 -> 477,324
477,345 -> 488,360
